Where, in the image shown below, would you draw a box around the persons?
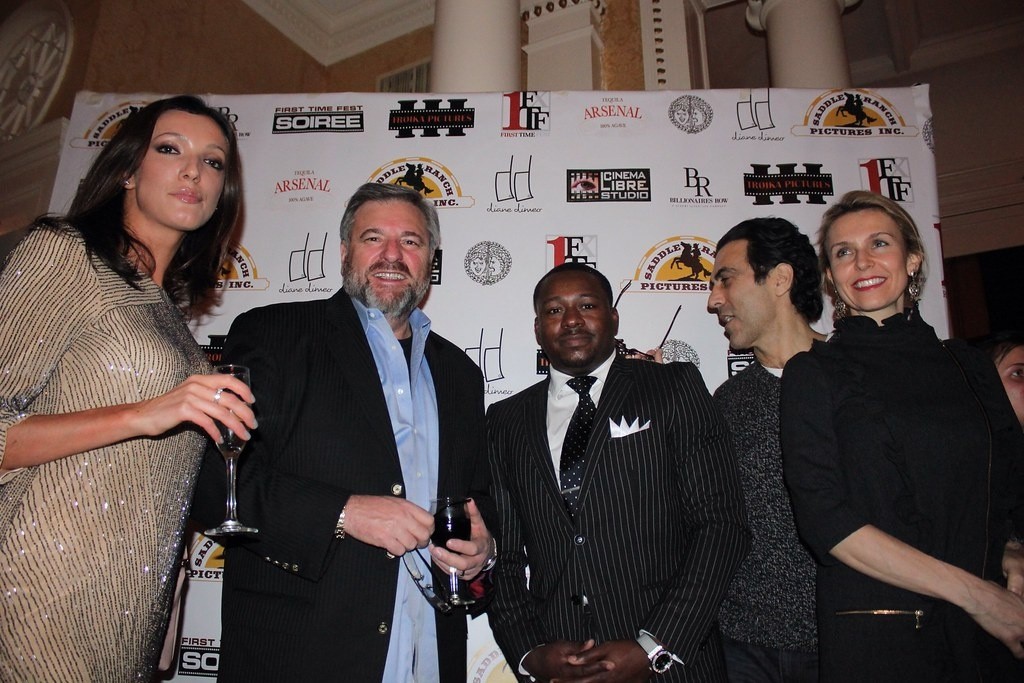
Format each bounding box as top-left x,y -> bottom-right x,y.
486,262 -> 751,683
0,97 -> 259,683
190,183 -> 500,683
780,190 -> 1024,683
625,215 -> 836,683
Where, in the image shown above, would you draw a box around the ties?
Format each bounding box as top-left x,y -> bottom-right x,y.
560,376 -> 599,521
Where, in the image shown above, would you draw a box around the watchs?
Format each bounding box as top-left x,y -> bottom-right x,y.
481,538 -> 497,571
636,633 -> 673,674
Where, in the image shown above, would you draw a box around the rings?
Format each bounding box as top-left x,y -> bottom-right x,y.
214,389 -> 222,404
459,570 -> 465,576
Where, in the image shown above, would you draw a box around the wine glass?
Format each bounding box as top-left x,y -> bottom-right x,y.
204,363 -> 259,535
427,496 -> 475,606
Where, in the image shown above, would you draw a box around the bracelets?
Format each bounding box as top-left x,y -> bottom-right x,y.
334,504 -> 345,539
